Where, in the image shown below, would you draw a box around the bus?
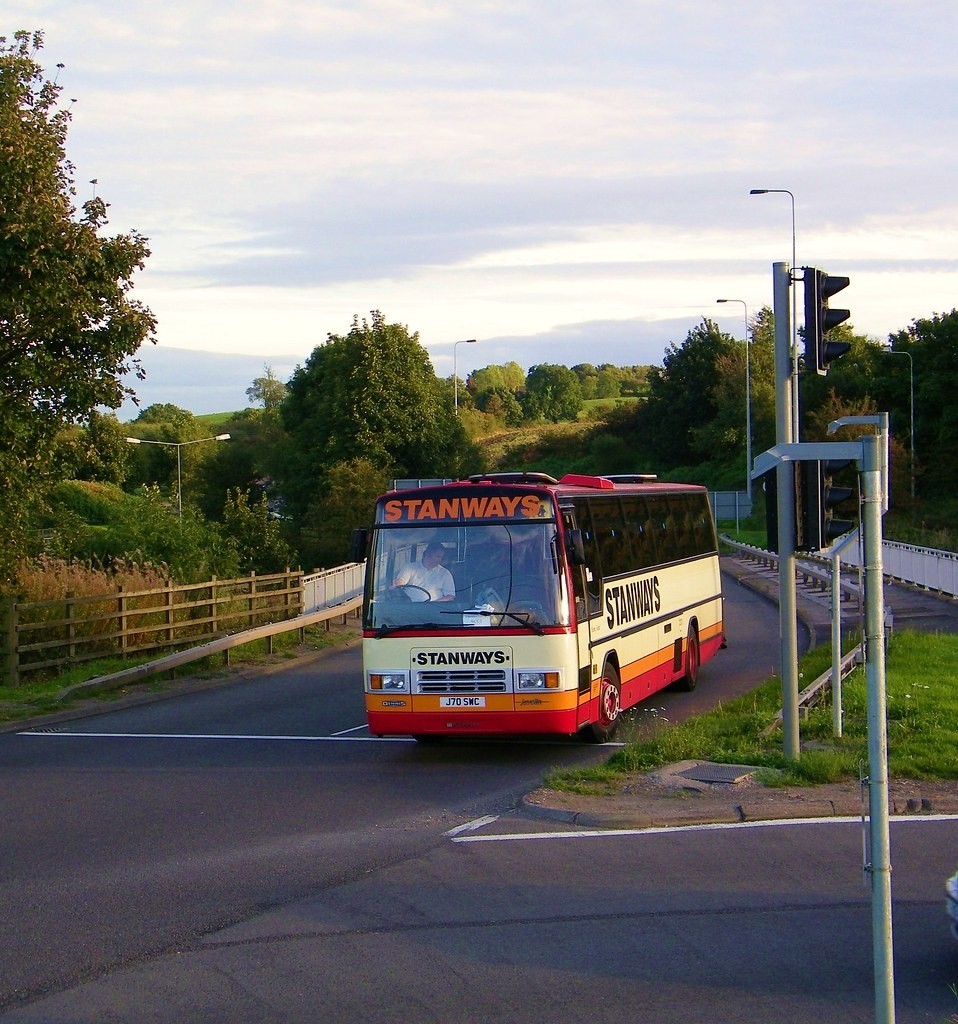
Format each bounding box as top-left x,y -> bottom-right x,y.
346,470 -> 726,745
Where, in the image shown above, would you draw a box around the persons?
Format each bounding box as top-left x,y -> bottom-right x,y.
389,542 -> 456,602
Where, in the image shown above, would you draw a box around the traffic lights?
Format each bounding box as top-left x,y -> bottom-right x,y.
821,457 -> 861,547
818,272 -> 852,369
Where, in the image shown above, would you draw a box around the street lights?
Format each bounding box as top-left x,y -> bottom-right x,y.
717,294 -> 753,570
878,344 -> 916,505
454,339 -> 478,415
750,187 -> 800,773
122,432 -> 232,543
